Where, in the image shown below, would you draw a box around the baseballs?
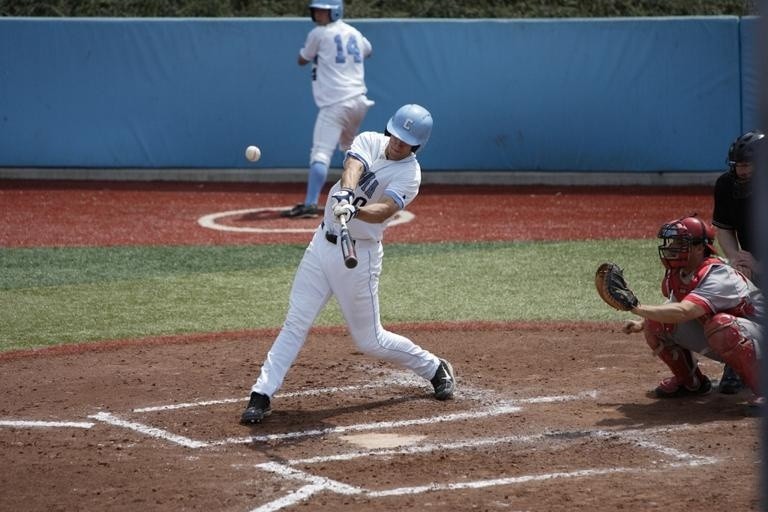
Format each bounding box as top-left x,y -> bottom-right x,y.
246,146 -> 261,162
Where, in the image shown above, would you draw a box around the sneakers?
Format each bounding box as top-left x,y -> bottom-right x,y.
719,361 -> 746,397
239,391 -> 273,426
654,375 -> 713,400
430,358 -> 457,401
280,203 -> 319,221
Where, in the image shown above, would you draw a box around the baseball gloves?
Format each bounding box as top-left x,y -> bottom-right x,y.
596,263 -> 638,312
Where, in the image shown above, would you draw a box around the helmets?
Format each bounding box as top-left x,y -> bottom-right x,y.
386,103 -> 434,155
726,129 -> 768,199
309,0 -> 344,24
663,215 -> 717,269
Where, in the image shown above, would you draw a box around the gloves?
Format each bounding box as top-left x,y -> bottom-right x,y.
330,189 -> 357,209
333,204 -> 362,224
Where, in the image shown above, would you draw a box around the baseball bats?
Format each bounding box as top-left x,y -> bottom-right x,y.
340,214 -> 357,268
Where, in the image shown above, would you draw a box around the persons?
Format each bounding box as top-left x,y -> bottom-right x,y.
594,214 -> 763,401
238,103 -> 455,424
282,0 -> 373,218
710,129 -> 768,394
